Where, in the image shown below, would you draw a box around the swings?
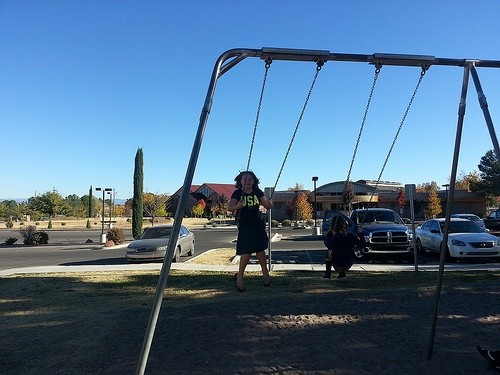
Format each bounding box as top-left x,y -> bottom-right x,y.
326,63 -> 430,249
235,58 -> 326,235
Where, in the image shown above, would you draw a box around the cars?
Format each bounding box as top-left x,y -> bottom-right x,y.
126,225 -> 195,263
395,209 -> 500,261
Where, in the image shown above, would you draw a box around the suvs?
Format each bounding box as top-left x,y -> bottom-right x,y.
322,208 -> 413,262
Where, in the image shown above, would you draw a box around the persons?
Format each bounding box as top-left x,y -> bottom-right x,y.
324,216 -> 367,278
228,170 -> 273,292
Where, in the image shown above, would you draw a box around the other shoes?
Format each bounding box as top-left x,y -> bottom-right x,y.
323,275 -> 331,278
337,275 -> 347,279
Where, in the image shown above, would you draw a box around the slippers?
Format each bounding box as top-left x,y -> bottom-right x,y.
263,272 -> 271,287
234,273 -> 246,292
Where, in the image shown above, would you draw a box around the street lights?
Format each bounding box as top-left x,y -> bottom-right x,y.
96,187 -> 112,244
312,176 -> 320,236
442,184 -> 450,205
